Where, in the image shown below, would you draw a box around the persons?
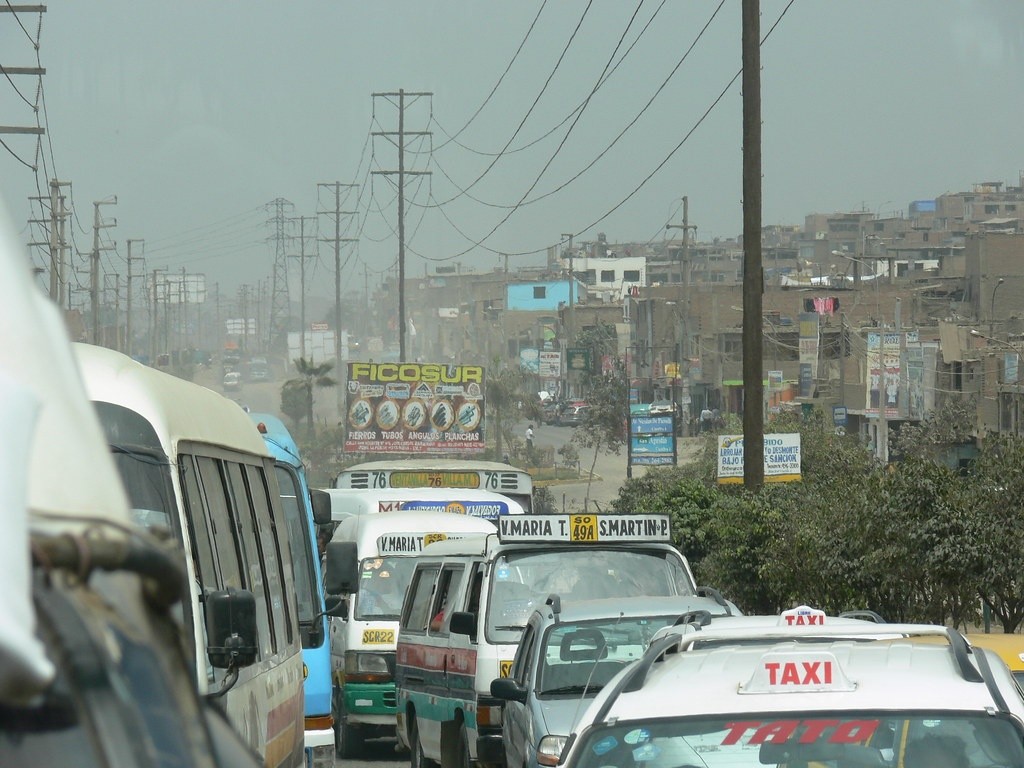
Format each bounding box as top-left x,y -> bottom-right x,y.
871,366 -> 900,407
525,425 -> 535,447
430,570 -> 483,632
903,733 -> 969,768
372,561 -> 412,614
687,405 -> 720,437
555,402 -> 566,426
626,565 -> 655,596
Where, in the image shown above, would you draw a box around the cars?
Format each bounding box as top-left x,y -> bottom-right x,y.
540,603 -> 1024,768
216,349 -> 266,390
538,400 -> 558,427
560,406 -> 593,426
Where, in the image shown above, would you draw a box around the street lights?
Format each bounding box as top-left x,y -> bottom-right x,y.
986,278 -> 1005,348
828,250 -> 888,468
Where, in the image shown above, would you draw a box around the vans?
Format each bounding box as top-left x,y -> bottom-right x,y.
319,508 -> 497,759
395,512 -> 706,768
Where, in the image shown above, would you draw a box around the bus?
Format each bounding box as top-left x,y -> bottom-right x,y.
247,412 -> 359,767
339,459 -> 539,514
71,339 -> 366,768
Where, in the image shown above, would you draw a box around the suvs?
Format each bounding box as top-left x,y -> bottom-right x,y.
489,586 -> 746,768
